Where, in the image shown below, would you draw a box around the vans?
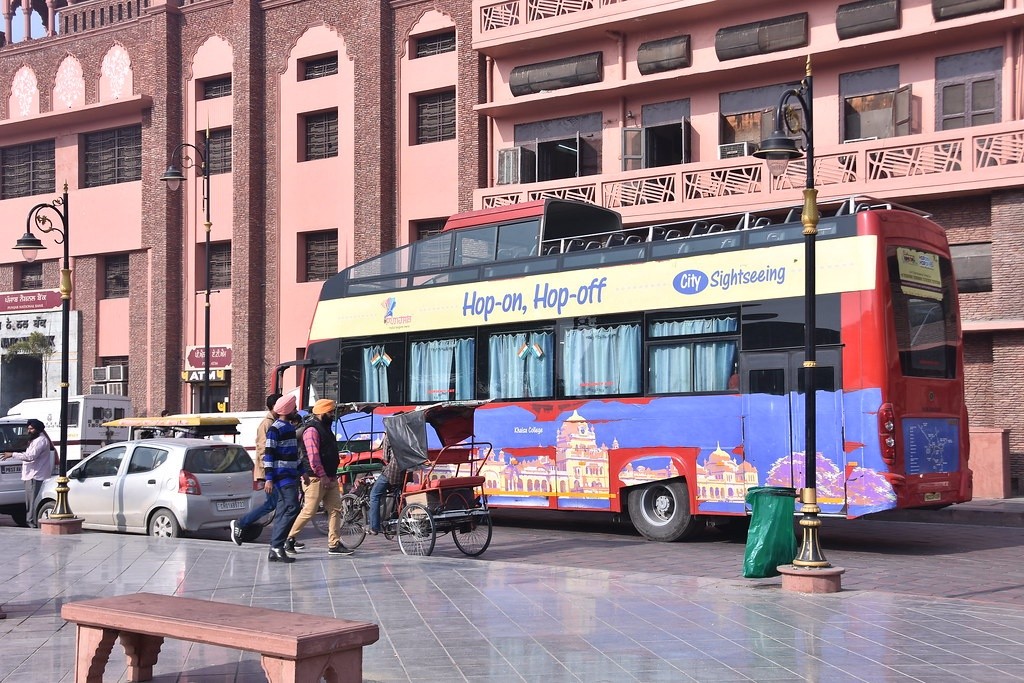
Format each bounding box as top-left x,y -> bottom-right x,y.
154,410 -> 269,468
0,418 -> 56,526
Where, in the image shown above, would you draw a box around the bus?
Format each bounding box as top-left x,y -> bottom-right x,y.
271,193 -> 973,541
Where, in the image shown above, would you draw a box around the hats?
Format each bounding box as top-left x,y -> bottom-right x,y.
274,394 -> 296,415
27,419 -> 45,432
312,398 -> 335,414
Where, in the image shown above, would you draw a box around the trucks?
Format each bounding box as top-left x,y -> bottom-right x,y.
7,394 -> 131,477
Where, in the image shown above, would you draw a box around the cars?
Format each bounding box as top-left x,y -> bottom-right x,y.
32,437 -> 269,540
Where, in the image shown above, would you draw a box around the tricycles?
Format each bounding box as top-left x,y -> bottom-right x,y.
249,403 -> 386,535
329,399 -> 493,557
101,413 -> 242,448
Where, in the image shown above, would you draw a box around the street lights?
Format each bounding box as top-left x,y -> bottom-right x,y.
160,107 -> 220,413
12,179 -> 84,534
750,53 -> 846,592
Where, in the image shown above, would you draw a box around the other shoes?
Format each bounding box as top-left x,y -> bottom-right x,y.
362,525 -> 378,535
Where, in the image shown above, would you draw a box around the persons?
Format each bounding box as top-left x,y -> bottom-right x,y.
264,395 -> 309,563
361,412 -> 407,536
229,394 -> 306,549
0,420 -> 51,528
140,431 -> 154,439
283,399 -> 355,555
289,414 -> 308,475
161,410 -> 171,417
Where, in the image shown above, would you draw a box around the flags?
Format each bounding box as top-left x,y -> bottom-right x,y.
530,344 -> 543,359
516,343 -> 530,359
382,353 -> 392,367
370,353 -> 381,367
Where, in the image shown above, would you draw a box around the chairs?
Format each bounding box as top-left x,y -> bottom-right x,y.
136,451 -> 153,469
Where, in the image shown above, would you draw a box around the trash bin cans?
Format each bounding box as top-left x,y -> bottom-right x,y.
742,487 -> 798,578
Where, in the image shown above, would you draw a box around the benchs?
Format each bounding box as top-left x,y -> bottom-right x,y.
336,432 -> 387,473
405,442 -> 493,492
61,592 -> 379,683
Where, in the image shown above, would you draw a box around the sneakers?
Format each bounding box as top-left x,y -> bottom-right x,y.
284,541 -> 305,549
284,545 -> 297,555
268,547 -> 296,563
230,520 -> 242,546
328,543 -> 354,555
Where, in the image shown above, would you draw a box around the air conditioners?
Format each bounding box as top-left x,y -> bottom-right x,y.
718,142 -> 758,159
